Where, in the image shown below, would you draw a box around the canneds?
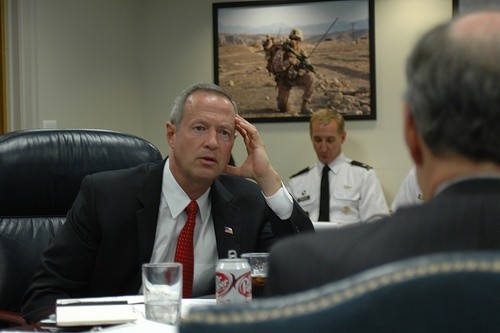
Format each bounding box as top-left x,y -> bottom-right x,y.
215,249 -> 252,305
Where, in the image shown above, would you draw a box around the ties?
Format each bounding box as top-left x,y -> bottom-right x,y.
318,164 -> 332,222
174,200 -> 198,299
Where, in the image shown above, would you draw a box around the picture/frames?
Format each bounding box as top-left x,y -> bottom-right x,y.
210,0 -> 377,125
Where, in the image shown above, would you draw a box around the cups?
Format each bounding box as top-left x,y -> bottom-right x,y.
142,262 -> 183,328
241,253 -> 269,298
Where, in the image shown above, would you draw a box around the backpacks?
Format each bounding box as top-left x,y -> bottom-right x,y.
265,42 -> 287,76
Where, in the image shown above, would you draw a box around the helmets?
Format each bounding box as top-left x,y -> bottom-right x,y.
289,29 -> 303,41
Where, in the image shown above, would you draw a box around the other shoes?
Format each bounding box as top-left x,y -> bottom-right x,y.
301,102 -> 313,114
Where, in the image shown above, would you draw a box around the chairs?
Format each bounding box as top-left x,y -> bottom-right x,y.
0,128 -> 163,333
177,248 -> 500,333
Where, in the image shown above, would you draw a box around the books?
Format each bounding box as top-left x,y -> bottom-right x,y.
55,299 -> 137,326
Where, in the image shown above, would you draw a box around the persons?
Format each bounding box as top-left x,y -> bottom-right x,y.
21,82 -> 316,324
392,166 -> 423,213
262,28 -> 315,113
265,6 -> 500,297
287,109 -> 390,225
